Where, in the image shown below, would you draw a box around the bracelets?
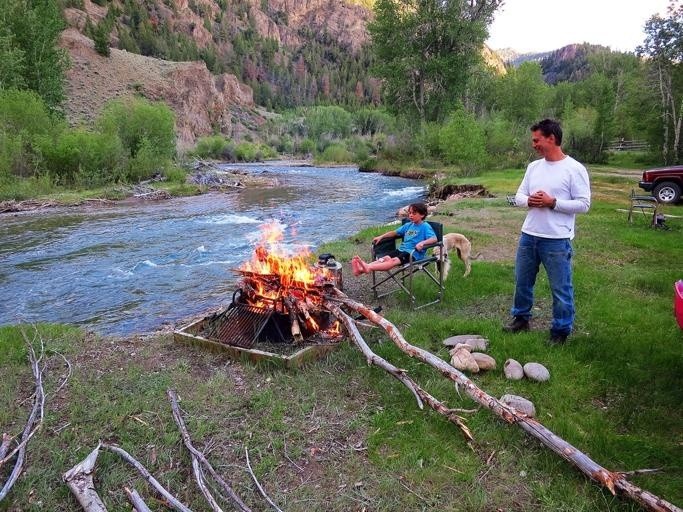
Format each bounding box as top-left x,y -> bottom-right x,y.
550,198 -> 556,209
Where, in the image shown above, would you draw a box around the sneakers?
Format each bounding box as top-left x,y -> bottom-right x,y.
546,333 -> 568,348
503,315 -> 529,332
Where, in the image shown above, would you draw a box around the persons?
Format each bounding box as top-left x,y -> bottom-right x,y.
503,120 -> 591,345
351,202 -> 437,276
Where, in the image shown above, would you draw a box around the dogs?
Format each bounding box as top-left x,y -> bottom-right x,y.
431,231 -> 483,284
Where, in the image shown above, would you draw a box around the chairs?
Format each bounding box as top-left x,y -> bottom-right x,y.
627,185 -> 659,225
371,218 -> 446,312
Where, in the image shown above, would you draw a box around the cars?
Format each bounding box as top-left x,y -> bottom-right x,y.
638,164 -> 683,205
